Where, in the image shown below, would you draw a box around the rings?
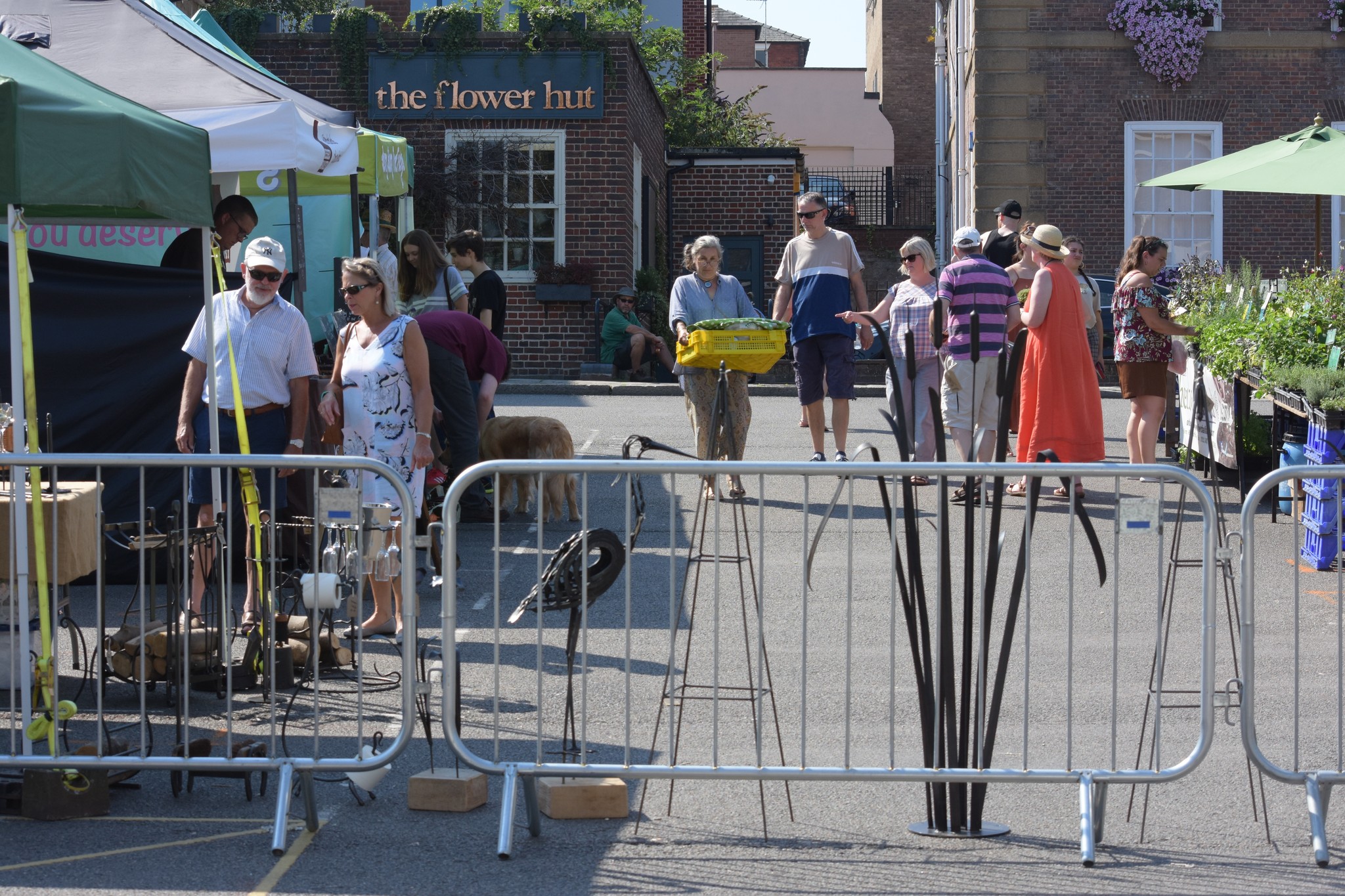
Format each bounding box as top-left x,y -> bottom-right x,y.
870,342 -> 873,343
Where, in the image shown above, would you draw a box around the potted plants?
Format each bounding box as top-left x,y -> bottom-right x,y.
519,6 -> 587,32
416,4 -> 483,32
1169,314 -> 1345,470
532,262 -> 595,301
219,7 -> 280,33
312,7 -> 379,32
637,291 -> 655,311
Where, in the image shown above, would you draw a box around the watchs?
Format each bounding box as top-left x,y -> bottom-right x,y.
288,439 -> 304,448
928,330 -> 935,337
416,432 -> 432,439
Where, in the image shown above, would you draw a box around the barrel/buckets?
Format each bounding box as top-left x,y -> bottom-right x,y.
362,502 -> 392,561
1277,424 -> 1308,515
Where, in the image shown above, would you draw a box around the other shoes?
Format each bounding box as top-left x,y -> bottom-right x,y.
834,451 -> 848,462
808,452 -> 826,462
630,368 -> 653,382
343,616 -> 419,644
480,478 -> 494,493
1126,477 -> 1175,483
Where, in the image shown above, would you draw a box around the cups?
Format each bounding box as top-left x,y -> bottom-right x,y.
512,248 -> 523,261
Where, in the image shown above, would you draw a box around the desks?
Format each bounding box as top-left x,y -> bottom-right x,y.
1234,375 -> 1308,523
253,379 -> 337,559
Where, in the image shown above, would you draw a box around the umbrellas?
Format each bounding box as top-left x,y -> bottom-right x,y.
1136,112 -> 1345,274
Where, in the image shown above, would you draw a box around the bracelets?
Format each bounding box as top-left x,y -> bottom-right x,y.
321,391 -> 336,403
1020,311 -> 1024,316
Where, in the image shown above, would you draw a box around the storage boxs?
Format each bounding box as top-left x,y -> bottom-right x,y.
1301,421 -> 1345,570
0,481 -> 104,586
677,329 -> 787,374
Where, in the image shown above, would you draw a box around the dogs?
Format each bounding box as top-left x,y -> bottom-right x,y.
479,416 -> 582,524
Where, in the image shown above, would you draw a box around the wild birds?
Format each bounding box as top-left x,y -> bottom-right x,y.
506,432 -> 704,786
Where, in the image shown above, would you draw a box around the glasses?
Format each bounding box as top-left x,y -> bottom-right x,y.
899,254 -> 921,265
796,208 -> 826,219
995,213 -> 1001,220
694,254 -> 722,267
1142,239 -> 1164,254
246,265 -> 283,282
230,214 -> 248,241
338,283 -> 371,297
1024,226 -> 1036,235
619,297 -> 635,304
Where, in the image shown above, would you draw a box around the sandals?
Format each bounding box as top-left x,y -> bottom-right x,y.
241,610 -> 262,636
799,419 -> 809,427
703,474 -> 747,500
909,447 -> 1085,504
173,609 -> 205,629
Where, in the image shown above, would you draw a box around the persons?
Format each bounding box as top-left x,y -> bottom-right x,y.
1110,235 -> 1202,482
361,209 -> 398,304
412,311 -> 511,526
599,287 -> 679,382
928,199 -> 1106,504
160,195 -> 258,278
669,235 -> 760,500
836,233 -> 948,485
772,192 -> 874,477
318,256 -> 434,643
394,229 -> 468,316
176,236 -> 319,626
445,229 -> 507,342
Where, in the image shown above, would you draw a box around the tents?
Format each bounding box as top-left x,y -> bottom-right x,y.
0,0 -> 414,760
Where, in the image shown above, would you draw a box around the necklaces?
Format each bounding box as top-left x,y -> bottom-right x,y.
700,278 -> 716,288
1031,268 -> 1034,272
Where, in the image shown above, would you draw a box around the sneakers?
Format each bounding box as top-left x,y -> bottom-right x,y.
460,497 -> 510,523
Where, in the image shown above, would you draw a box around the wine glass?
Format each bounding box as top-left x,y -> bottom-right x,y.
333,525 -> 346,575
361,518 -> 373,574
24,418 -> 43,473
374,524 -> 390,581
346,525 -> 359,581
385,521 -> 401,577
0,403 -> 12,454
321,522 -> 337,574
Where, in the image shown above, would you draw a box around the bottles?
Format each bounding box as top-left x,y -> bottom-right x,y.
854,324 -> 861,349
496,247 -> 503,260
484,247 -> 495,263
0,406 -> 13,452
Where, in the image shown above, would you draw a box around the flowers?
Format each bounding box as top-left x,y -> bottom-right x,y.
1318,0 -> 1345,40
1106,0 -> 1225,91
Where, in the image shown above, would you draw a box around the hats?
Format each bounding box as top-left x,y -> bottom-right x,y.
953,200 -> 1070,259
612,287 -> 640,304
245,236 -> 286,273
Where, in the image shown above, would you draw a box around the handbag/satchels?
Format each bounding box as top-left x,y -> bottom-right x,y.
1167,317 -> 1188,377
320,321 -> 360,446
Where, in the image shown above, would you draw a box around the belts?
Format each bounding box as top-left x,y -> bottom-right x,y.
204,402 -> 281,418
